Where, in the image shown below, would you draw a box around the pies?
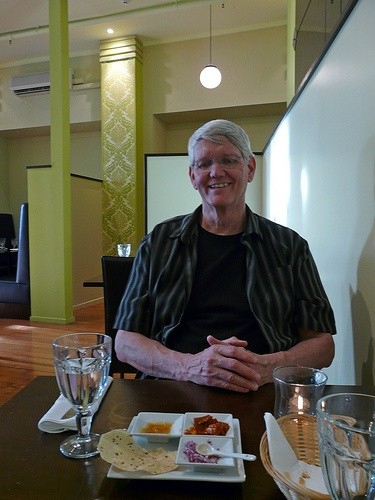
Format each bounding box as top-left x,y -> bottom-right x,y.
97,428 -> 182,475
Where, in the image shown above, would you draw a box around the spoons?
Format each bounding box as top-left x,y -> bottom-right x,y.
196,443 -> 256,461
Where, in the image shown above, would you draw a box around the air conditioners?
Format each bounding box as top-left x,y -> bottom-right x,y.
9,68 -> 74,96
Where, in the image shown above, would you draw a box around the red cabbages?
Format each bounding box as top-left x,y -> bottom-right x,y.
184,440 -> 221,464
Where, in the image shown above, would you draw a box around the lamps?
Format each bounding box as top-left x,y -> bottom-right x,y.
198,65 -> 221,89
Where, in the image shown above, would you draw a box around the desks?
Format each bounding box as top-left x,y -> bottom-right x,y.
0,249 -> 17,275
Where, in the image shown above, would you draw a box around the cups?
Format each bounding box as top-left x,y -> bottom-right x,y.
317,393 -> 375,500
0,238 -> 6,247
0,247 -> 5,253
118,244 -> 131,257
273,365 -> 328,419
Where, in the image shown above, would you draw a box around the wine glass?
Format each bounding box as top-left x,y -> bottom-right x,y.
52,332 -> 112,458
11,240 -> 18,249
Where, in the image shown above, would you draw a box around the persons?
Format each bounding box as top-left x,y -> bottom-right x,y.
112,119 -> 337,393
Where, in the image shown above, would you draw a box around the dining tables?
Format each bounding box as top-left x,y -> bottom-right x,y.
0,375 -> 374,499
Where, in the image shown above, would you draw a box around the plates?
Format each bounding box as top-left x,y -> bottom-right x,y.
107,412 -> 245,481
10,249 -> 19,250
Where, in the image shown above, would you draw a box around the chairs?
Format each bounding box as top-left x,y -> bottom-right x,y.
0,201 -> 30,305
102,256 -> 139,379
